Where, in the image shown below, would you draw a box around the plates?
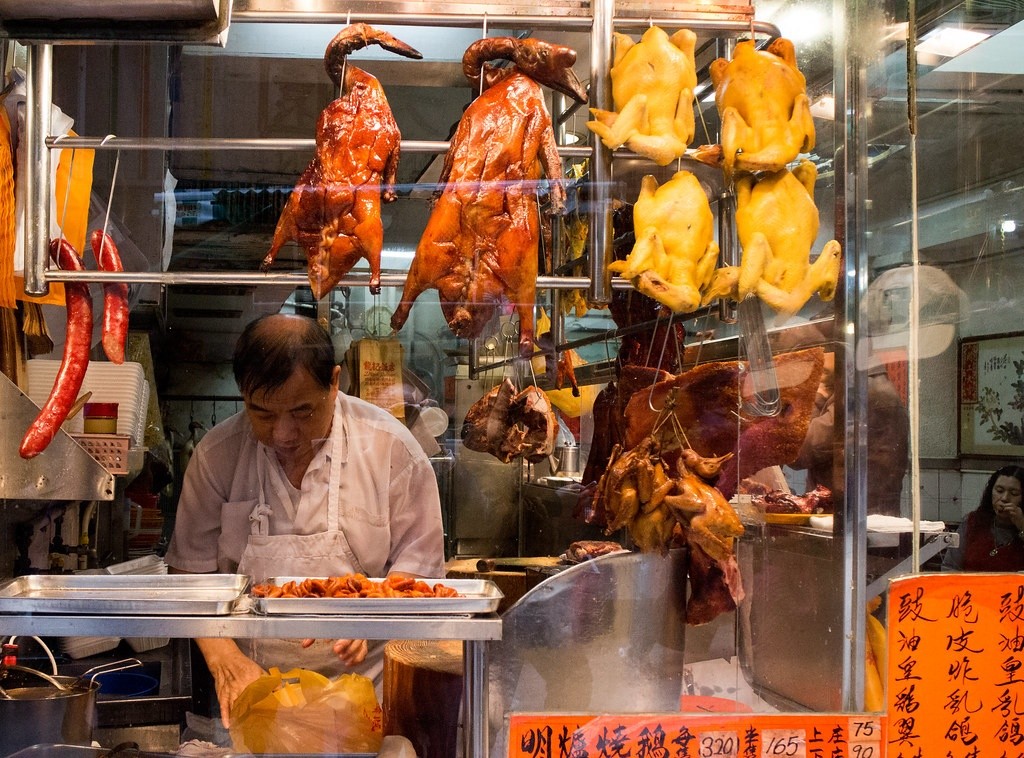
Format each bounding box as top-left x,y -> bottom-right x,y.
759,513 -> 834,525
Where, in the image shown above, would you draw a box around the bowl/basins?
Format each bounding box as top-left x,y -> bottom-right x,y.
80,672 -> 159,696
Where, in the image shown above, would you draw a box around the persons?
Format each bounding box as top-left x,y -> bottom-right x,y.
784,304 -> 908,565
940,464 -> 1024,579
164,312 -> 447,732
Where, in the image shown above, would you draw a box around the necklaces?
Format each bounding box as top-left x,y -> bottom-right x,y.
989,510 -> 1017,557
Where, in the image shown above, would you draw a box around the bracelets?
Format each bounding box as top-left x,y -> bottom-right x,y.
1018,530 -> 1024,538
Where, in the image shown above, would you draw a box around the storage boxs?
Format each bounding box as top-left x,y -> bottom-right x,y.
57,553 -> 171,659
68,433 -> 137,474
128,446 -> 149,472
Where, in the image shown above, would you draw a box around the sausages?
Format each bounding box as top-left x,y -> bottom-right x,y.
20,239 -> 94,458
91,230 -> 128,363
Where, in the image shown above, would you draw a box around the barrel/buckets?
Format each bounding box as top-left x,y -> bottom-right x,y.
555,441 -> 581,472
0,676 -> 102,758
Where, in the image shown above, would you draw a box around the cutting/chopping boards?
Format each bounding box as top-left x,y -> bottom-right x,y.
383,639 -> 465,758
